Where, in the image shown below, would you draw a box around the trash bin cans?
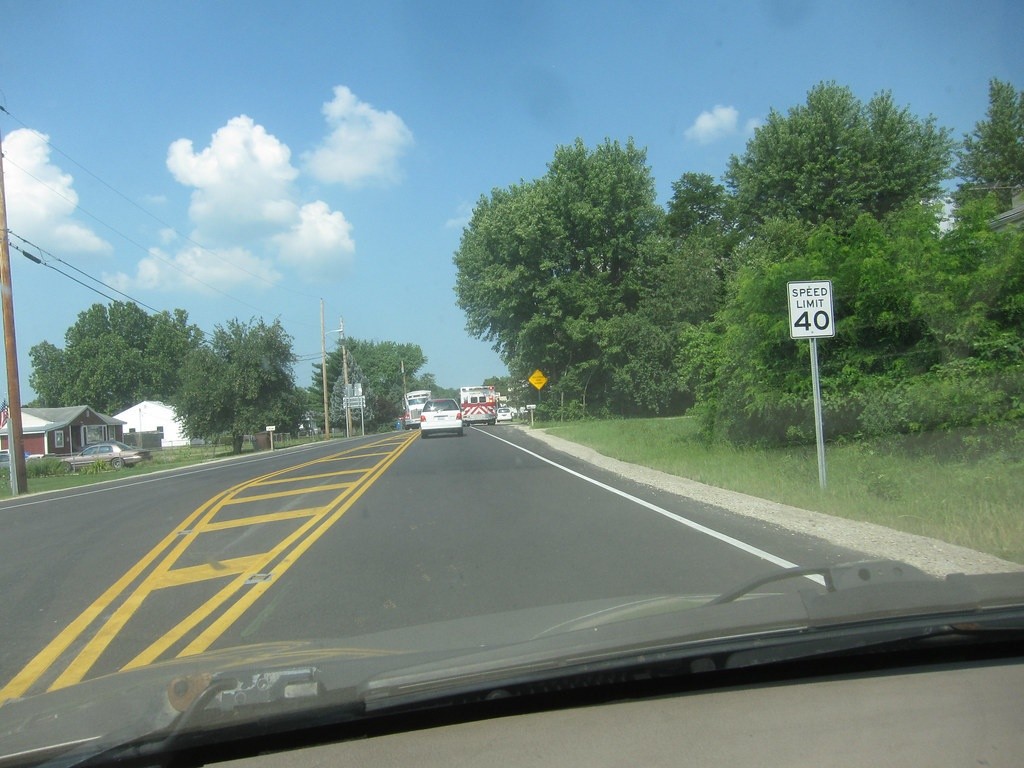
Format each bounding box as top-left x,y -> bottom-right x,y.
255,430 -> 271,451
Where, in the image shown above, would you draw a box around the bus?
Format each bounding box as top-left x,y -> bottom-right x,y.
402,389 -> 433,430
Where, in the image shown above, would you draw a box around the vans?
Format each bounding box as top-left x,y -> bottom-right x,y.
497,408 -> 513,422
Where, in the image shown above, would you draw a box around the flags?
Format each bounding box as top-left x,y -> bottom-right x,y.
0,393 -> 9,428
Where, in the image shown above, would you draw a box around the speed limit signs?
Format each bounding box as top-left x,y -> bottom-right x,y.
786,278 -> 835,339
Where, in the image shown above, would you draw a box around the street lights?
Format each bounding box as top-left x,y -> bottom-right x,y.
320,329 -> 344,441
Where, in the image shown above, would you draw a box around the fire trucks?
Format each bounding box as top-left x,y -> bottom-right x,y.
459,386 -> 497,427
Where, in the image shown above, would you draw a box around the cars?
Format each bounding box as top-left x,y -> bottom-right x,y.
0,450 -> 11,471
62,441 -> 150,472
27,453 -> 57,460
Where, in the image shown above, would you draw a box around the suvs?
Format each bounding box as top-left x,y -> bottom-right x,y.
421,398 -> 462,437
